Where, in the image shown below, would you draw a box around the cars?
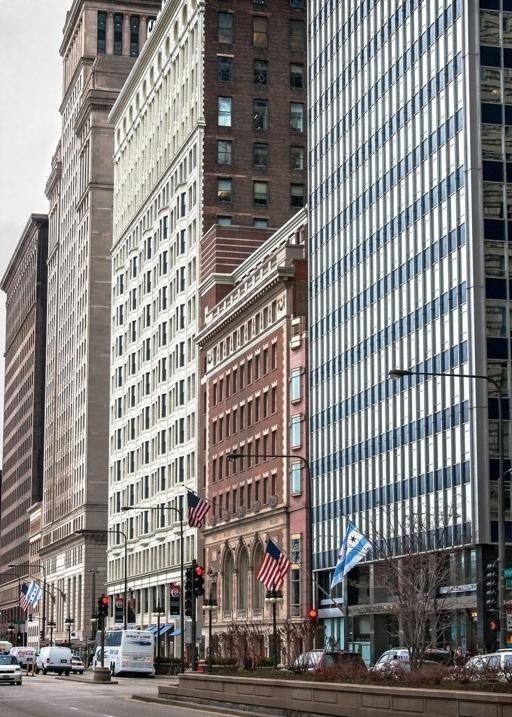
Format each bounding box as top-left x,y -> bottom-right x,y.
0,654 -> 24,686
72,655 -> 86,674
372,646 -> 511,683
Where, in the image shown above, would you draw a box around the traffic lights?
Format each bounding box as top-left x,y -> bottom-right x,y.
308,609 -> 318,618
98,594 -> 110,617
192,561 -> 206,597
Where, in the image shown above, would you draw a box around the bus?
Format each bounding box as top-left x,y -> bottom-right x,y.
91,627 -> 158,677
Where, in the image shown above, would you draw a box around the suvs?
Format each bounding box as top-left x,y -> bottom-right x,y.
285,646 -> 370,679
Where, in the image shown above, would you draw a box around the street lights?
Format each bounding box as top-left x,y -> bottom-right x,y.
201,592 -> 219,672
64,611 -> 74,648
390,369 -> 508,651
7,564 -> 46,649
152,597 -> 166,675
7,621 -> 15,644
120,505 -> 186,673
226,452 -> 315,650
263,580 -> 284,673
46,616 -> 57,646
75,529 -> 129,629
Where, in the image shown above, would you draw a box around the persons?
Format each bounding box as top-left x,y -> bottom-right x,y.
454,650 -> 467,666
465,648 -> 475,661
445,644 -> 455,666
388,655 -> 400,677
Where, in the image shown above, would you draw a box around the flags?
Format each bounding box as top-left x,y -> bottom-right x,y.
19,583 -> 31,612
256,538 -> 292,593
329,521 -> 373,591
26,578 -> 43,607
187,490 -> 212,527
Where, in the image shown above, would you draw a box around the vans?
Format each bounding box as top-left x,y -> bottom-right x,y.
10,646 -> 37,671
33,646 -> 73,675
